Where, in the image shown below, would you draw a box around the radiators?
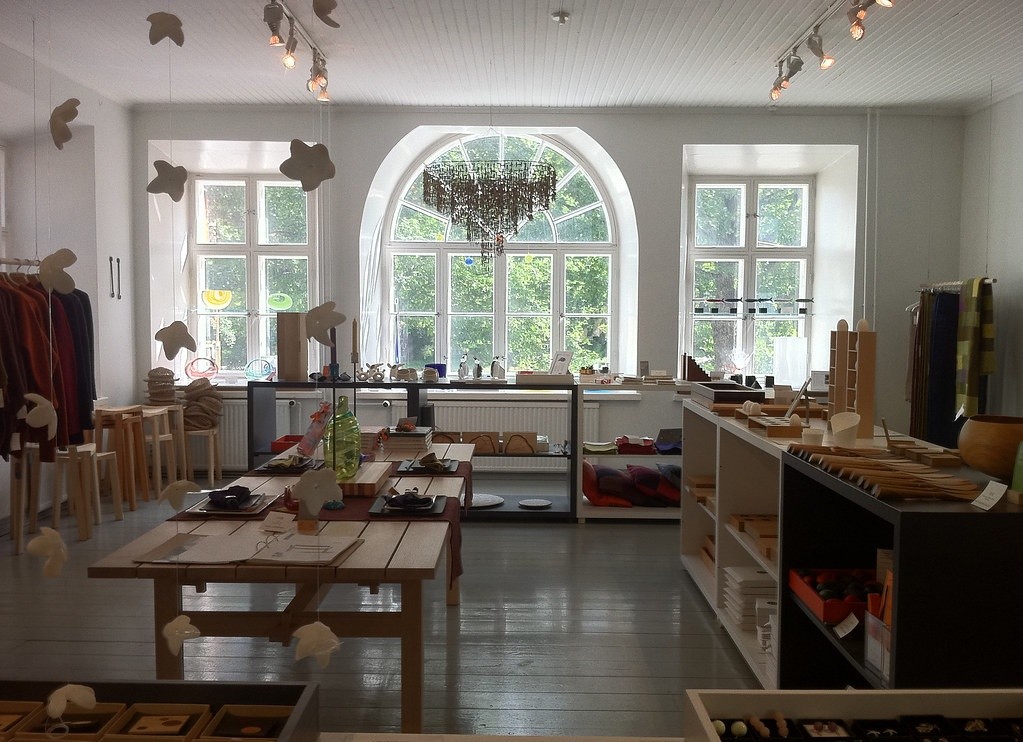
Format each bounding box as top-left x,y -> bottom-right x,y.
146,399 -> 302,472
391,401 -> 601,473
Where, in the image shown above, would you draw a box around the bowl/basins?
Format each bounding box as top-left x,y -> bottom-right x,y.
957,416 -> 1023,474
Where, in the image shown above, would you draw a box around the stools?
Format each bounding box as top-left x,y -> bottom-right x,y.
10,404 -> 225,556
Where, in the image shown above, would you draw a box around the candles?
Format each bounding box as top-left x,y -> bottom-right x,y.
330,327 -> 337,363
351,317 -> 357,352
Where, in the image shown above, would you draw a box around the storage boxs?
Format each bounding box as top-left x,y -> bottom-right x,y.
690,390 -> 718,409
0,701 -> 296,742
516,371 -> 574,385
690,382 -> 766,403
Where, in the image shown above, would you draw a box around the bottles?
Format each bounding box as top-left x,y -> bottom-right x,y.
322,396 -> 361,479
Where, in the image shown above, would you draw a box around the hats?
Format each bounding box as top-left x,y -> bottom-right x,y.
144,367 -> 181,406
182,378 -> 224,430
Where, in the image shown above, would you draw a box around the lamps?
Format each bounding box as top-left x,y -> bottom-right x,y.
262,0 -> 331,102
767,0 -> 895,102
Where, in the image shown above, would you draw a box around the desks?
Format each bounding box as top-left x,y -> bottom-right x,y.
87,443 -> 476,735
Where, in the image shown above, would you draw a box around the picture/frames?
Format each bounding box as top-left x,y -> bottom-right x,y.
549,351 -> 574,376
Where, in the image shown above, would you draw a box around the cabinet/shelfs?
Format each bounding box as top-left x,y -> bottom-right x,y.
678,398 -> 945,691
247,381 -> 578,524
777,450 -> 1023,690
846,319 -> 878,438
827,319 -> 848,432
578,384 -> 816,523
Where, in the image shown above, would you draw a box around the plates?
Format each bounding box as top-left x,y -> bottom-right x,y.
519,498 -> 552,508
460,491 -> 505,507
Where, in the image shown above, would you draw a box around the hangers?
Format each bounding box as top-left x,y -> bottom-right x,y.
0,257 -> 41,287
905,280 -> 966,312
